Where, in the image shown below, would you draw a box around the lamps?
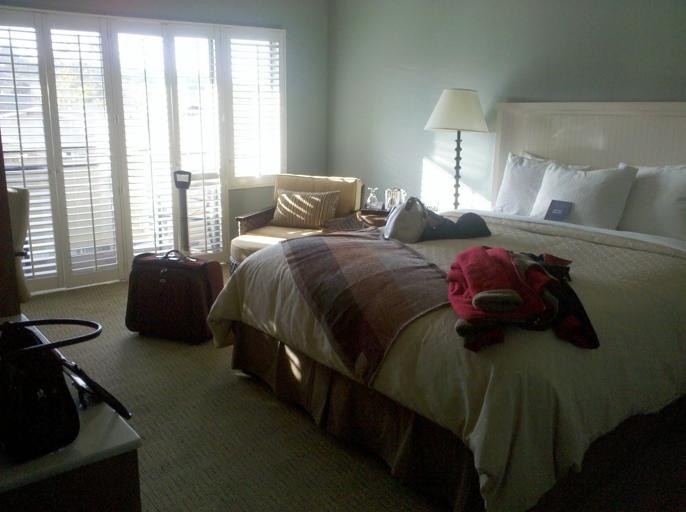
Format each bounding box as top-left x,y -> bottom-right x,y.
423,89 -> 490,210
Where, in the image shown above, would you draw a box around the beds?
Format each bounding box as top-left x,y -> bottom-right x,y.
207,101 -> 686,512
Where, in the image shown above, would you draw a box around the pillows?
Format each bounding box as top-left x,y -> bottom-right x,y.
618,164 -> 686,238
492,152 -> 590,217
270,190 -> 340,229
528,163 -> 637,230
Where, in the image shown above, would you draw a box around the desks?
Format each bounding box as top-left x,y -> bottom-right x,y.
0,313 -> 143,512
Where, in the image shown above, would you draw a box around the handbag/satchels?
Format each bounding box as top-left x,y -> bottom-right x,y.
384,197 -> 425,243
0,319 -> 132,462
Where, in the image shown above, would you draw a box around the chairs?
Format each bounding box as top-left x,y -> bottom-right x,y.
229,175 -> 361,274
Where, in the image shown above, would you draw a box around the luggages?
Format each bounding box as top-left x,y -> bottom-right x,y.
125,250 -> 223,339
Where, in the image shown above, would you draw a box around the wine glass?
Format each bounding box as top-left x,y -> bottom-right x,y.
366,187 -> 378,208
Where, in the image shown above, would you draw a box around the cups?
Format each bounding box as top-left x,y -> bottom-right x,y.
401,187 -> 408,205
392,188 -> 400,208
385,187 -> 391,210
